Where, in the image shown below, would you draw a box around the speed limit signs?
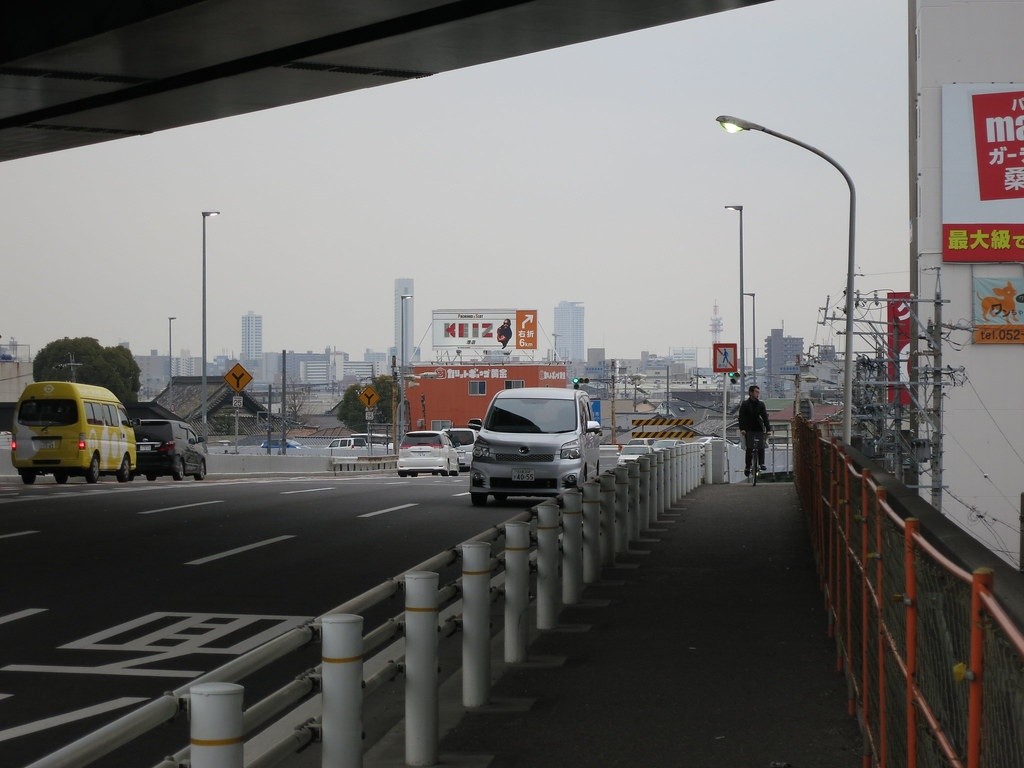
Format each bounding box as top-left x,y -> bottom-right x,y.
232,396 -> 245,408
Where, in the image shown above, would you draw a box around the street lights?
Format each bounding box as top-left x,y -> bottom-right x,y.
169,316 -> 177,417
742,293 -> 758,387
723,204 -> 747,406
200,210 -> 222,451
401,294 -> 414,437
715,111 -> 859,448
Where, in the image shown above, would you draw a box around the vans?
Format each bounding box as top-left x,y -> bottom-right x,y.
10,380 -> 141,486
468,387 -> 602,504
131,419 -> 207,480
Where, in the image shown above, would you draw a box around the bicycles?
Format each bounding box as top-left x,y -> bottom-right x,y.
742,430 -> 772,486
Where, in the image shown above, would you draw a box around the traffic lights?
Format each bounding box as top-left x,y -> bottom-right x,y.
572,378 -> 590,383
572,383 -> 579,389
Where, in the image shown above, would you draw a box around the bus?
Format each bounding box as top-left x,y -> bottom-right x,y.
351,433 -> 393,450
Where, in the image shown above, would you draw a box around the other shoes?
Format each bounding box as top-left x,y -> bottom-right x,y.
745,465 -> 750,476
761,464 -> 767,471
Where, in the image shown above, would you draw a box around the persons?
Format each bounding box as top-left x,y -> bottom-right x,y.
497,318 -> 513,349
739,386 -> 772,477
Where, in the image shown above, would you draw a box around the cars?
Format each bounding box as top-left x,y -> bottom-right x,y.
325,437 -> 368,449
262,438 -> 310,448
395,431 -> 461,479
440,427 -> 479,469
